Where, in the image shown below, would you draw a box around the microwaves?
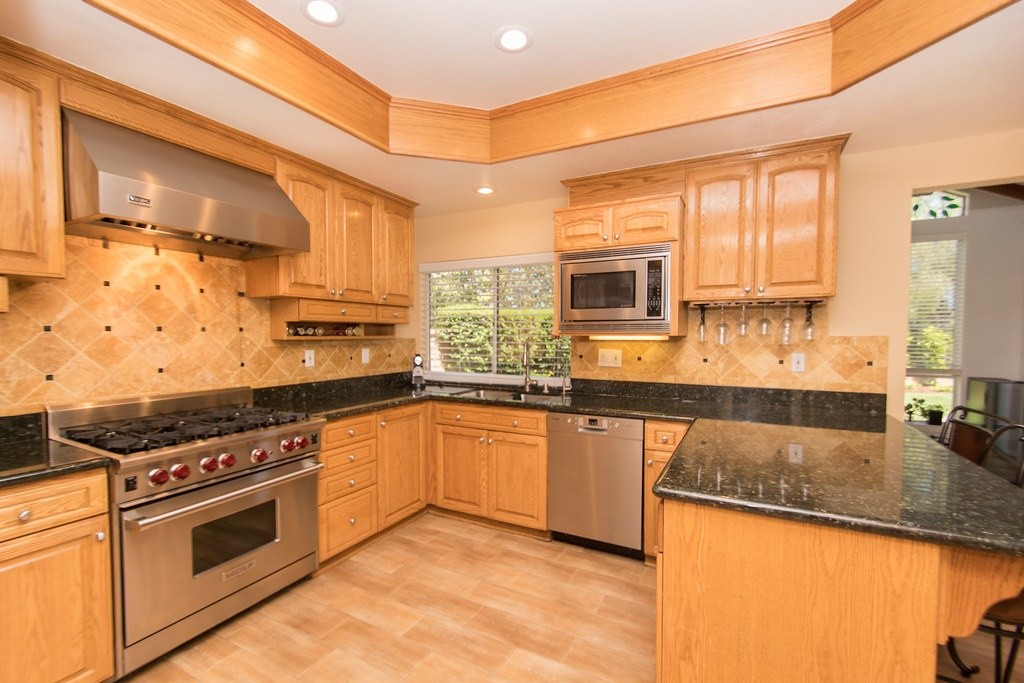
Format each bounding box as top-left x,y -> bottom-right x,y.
559,242 -> 672,335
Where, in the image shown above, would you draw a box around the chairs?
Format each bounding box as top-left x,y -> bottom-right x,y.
937,406 -> 1024,683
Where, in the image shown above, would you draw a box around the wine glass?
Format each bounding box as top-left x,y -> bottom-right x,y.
714,303 -> 729,347
778,302 -> 797,347
697,304 -> 708,344
801,306 -> 816,341
736,302 -> 750,337
756,303 -> 772,336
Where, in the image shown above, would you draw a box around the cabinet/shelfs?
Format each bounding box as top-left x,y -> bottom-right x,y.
275,157 -> 415,340
644,418 -> 689,556
0,51 -> 64,276
432,402 -> 547,530
319,412 -> 380,562
554,150 -> 839,297
377,403 -> 429,530
1,467 -> 115,683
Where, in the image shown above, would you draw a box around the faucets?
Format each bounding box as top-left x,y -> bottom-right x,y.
523,342 -> 538,392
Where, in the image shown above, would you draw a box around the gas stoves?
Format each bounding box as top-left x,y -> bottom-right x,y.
45,385 -> 327,505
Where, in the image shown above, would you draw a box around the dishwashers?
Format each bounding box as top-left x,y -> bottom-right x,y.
546,412 -> 645,562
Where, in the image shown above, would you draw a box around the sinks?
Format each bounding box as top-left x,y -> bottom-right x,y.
501,393 -> 572,406
455,389 -> 516,400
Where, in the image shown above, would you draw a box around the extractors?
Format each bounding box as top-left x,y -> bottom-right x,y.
61,105 -> 310,261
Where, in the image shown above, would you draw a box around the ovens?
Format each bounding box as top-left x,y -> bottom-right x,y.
109,449 -> 319,680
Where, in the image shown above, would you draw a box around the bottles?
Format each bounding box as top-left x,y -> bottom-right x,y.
286,325 -> 361,336
